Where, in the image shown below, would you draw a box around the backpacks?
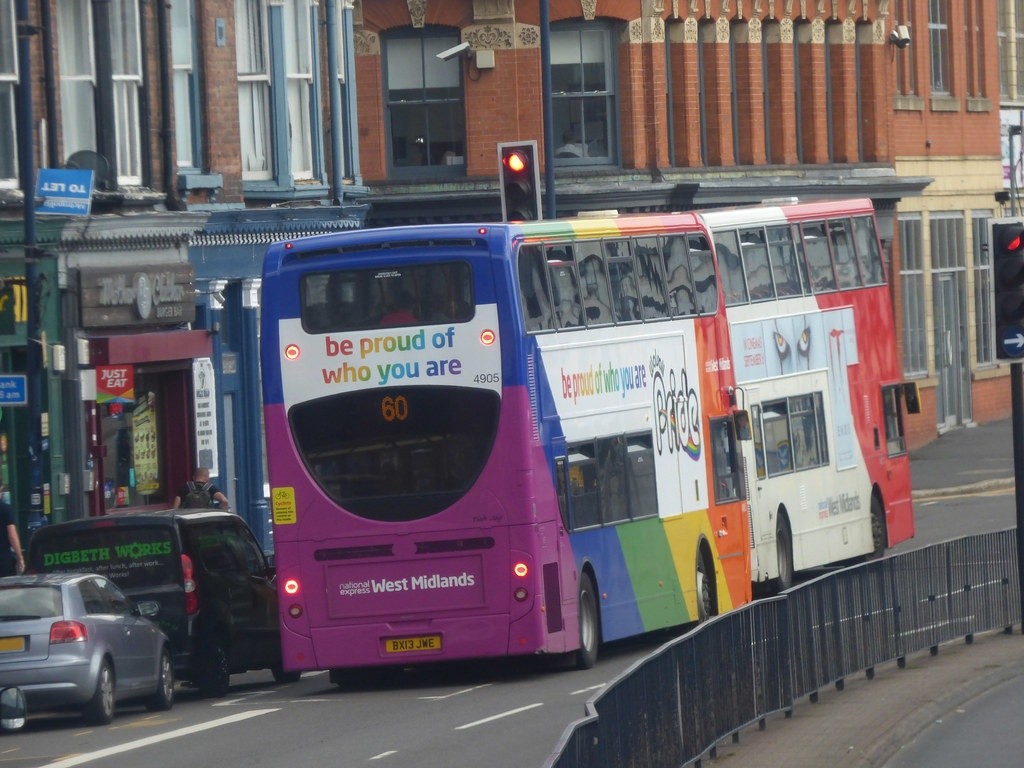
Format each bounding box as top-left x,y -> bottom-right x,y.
184,480 -> 215,509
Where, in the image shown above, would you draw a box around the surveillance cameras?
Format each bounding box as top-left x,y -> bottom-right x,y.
897,25 -> 910,45
436,41 -> 472,62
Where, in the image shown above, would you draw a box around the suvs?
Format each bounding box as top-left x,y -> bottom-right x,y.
25,507 -> 301,699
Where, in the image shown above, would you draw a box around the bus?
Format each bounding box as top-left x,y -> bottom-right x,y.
254,197 -> 916,690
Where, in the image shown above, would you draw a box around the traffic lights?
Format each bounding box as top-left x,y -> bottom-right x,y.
987,217 -> 1024,364
496,140 -> 543,224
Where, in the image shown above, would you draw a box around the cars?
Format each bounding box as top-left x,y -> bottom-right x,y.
0,572 -> 176,729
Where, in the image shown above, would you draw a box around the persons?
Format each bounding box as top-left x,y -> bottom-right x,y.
173,467 -> 229,510
377,291 -> 421,325
0,477 -> 25,576
